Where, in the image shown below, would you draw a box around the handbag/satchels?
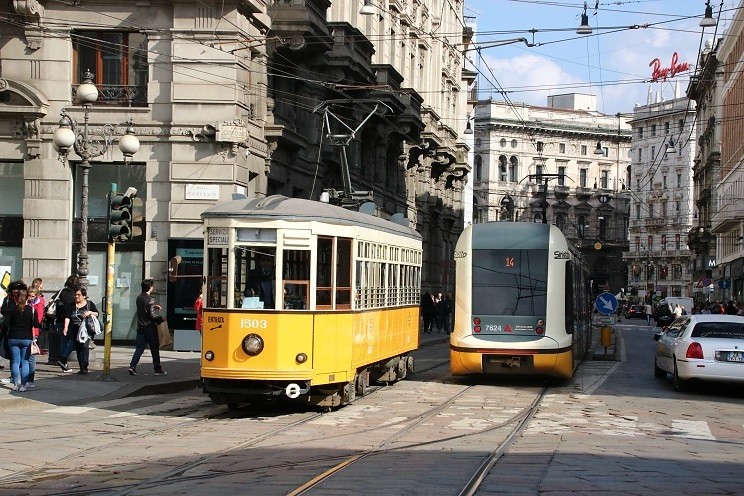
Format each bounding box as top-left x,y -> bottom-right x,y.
31,340 -> 41,355
87,315 -> 101,335
47,303 -> 56,318
0,342 -> 11,359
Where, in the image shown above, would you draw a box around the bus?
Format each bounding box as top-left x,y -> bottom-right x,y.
449,172 -> 594,385
194,98 -> 423,413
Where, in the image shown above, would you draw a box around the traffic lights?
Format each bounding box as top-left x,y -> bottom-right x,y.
107,193 -> 132,244
130,195 -> 143,241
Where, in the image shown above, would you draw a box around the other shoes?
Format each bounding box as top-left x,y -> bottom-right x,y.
25,382 -> 34,388
154,370 -> 167,375
58,360 -> 72,372
77,369 -> 88,373
13,386 -> 27,392
129,366 -> 138,375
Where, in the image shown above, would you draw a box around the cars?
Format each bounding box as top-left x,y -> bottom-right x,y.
653,313 -> 744,394
622,296 -> 693,336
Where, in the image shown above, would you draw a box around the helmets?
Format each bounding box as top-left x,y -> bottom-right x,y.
660,299 -> 666,305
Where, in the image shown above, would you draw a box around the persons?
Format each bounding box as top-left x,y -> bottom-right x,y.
691,300 -> 744,316
243,250 -> 293,309
646,302 -> 651,325
421,292 -> 455,334
0,278 -> 45,391
52,275 -> 79,373
591,300 -> 595,313
654,299 -> 671,327
668,303 -> 687,320
617,302 -> 623,322
63,287 -> 99,374
194,286 -> 202,335
129,279 -> 167,375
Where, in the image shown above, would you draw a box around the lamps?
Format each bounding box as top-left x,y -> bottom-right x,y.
698,4 -> 718,29
573,9 -> 593,36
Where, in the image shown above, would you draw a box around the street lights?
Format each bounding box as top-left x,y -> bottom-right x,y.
50,67 -> 141,301
